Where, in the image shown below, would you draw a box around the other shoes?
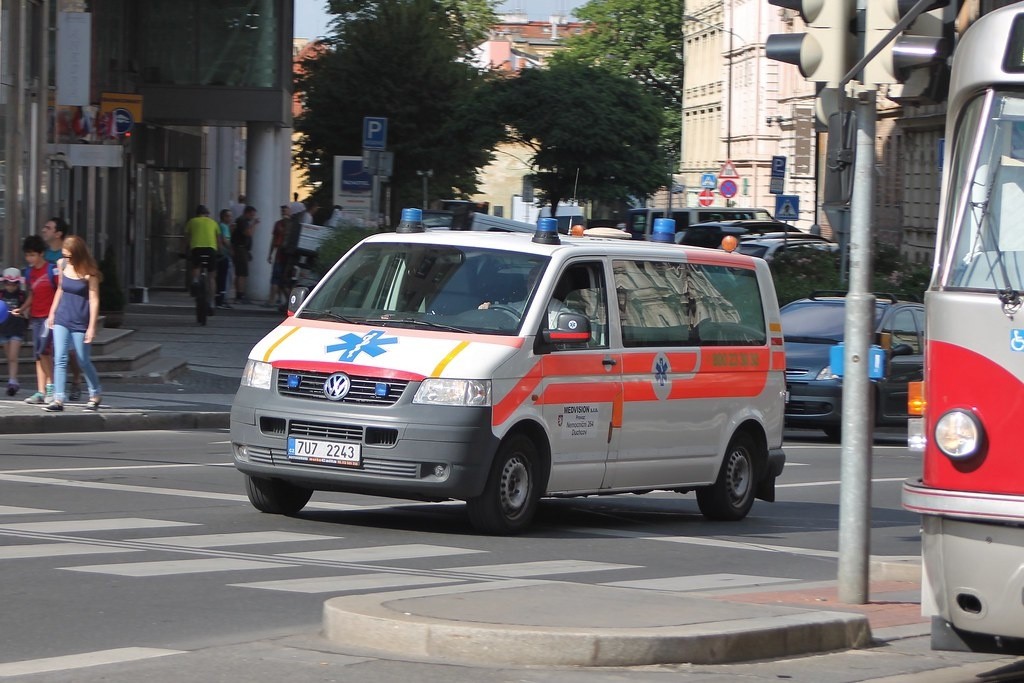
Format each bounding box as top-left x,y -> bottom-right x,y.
42,402 -> 64,412
191,282 -> 198,297
83,400 -> 99,412
208,307 -> 214,316
7,384 -> 17,395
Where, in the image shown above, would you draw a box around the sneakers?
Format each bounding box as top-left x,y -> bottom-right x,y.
24,392 -> 44,403
44,384 -> 55,404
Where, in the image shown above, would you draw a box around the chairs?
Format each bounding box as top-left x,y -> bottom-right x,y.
565,288 -> 606,347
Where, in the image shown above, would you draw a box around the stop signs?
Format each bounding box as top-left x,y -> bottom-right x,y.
700,191 -> 714,206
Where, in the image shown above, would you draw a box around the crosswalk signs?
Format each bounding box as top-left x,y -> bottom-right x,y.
776,193 -> 799,221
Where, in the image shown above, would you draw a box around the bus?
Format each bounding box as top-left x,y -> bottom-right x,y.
891,1 -> 1024,656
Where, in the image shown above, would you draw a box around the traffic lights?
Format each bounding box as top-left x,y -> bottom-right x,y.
764,0 -> 858,84
862,0 -> 946,88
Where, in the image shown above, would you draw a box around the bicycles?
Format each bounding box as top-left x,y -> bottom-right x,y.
179,250 -> 222,327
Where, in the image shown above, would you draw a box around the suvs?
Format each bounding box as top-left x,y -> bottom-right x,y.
423,194 -> 849,309
779,289 -> 925,438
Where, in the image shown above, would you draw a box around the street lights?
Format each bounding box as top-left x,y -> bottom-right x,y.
681,12 -> 747,209
416,167 -> 433,209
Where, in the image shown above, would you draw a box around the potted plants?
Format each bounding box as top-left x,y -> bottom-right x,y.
99,242 -> 129,329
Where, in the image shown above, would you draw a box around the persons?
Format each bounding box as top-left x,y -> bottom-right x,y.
477,267 -> 579,330
181,193 -> 392,315
0,218 -> 103,412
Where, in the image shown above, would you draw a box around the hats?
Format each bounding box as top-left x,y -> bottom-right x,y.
197,205 -> 209,215
0,267 -> 25,285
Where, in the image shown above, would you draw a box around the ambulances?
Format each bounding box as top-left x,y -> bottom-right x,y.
230,206 -> 788,536
